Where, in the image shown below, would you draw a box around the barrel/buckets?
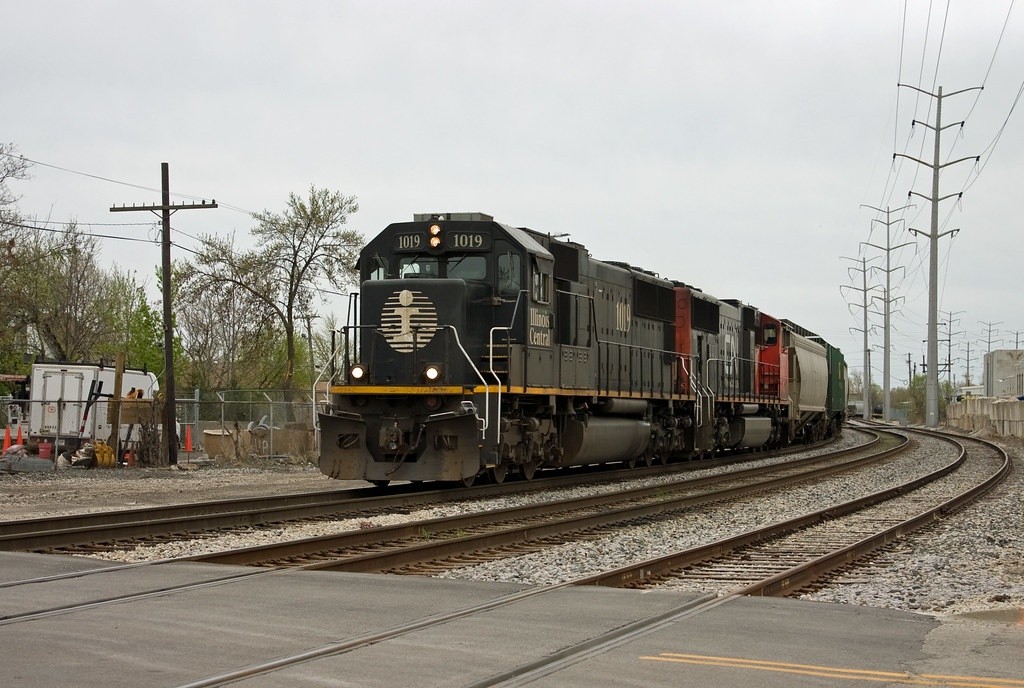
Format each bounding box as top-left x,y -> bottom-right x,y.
39,442 -> 52,459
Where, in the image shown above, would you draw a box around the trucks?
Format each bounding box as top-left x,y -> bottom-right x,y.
27,363 -> 181,457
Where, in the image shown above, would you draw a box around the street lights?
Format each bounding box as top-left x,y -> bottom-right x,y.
293,312 -> 322,403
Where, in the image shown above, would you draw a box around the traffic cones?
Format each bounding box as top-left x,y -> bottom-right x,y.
2,424 -> 12,457
16,426 -> 23,445
181,424 -> 193,452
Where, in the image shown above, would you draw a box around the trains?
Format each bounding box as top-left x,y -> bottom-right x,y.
318,211 -> 851,490
